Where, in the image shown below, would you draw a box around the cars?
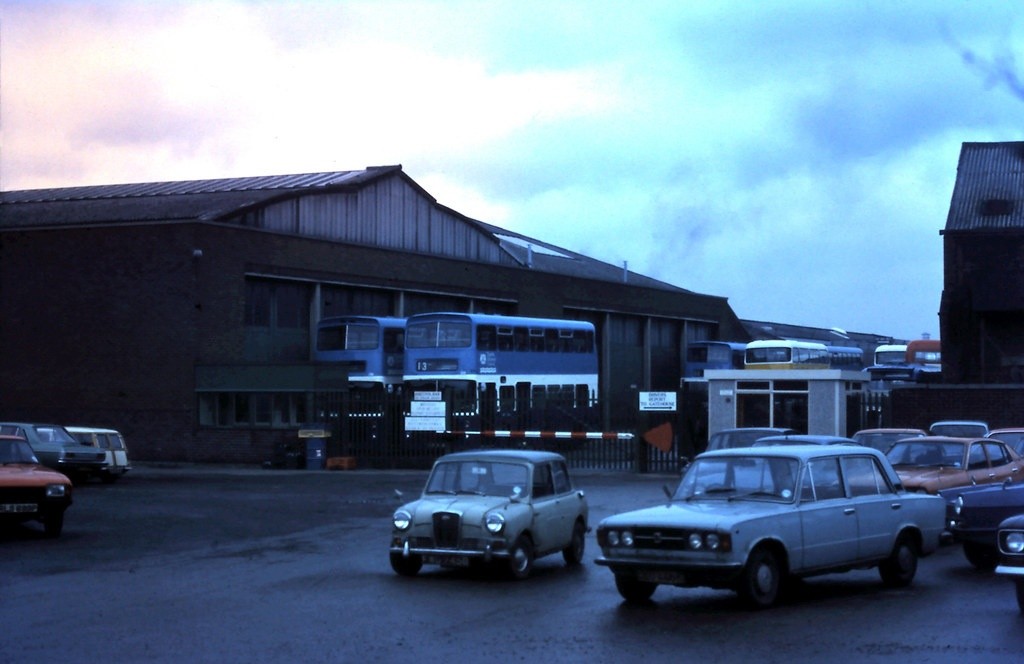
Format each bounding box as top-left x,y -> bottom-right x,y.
704,427 -> 794,453
828,435 -> 1024,496
986,429 -> 1023,454
0,422 -> 109,480
388,448 -> 592,582
851,429 -> 926,464
995,514 -> 1024,612
938,475 -> 1024,570
593,444 -> 953,615
929,422 -> 990,457
679,435 -> 876,495
0,434 -> 74,534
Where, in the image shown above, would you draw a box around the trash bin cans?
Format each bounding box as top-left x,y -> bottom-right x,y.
301,423 -> 334,469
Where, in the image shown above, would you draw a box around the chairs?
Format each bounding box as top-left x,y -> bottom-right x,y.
914,451 -> 990,468
451,480 -> 552,498
705,480 -> 862,506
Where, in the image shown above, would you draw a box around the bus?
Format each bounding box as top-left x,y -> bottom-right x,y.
311,315 -> 463,432
401,313 -> 600,432
681,337 -> 942,384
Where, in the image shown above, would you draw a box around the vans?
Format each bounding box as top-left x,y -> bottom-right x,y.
36,428 -> 132,481
